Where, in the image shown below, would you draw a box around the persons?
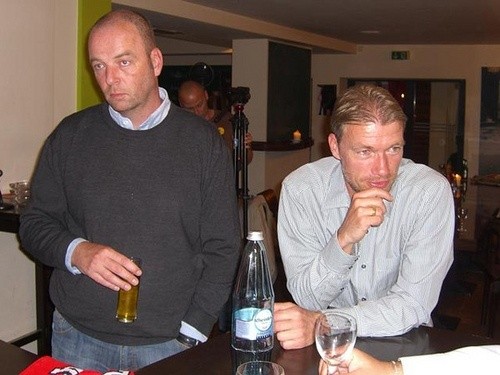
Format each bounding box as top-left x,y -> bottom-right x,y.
317,343 -> 500,375
178,79 -> 252,165
16,9 -> 241,373
262,83 -> 454,349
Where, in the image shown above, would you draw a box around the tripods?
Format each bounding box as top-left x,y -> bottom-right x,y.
233,102 -> 251,242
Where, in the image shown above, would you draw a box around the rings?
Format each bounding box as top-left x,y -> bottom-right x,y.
370,207 -> 377,217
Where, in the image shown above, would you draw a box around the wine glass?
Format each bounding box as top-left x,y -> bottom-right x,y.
314,310 -> 357,375
456,207 -> 468,232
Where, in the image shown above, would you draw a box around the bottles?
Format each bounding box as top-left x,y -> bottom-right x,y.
231,230 -> 276,375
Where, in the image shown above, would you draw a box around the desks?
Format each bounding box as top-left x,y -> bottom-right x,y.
0,193 -> 53,355
136,330 -> 500,375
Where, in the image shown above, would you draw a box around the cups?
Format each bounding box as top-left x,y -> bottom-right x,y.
236,360 -> 285,375
116,256 -> 141,324
15,181 -> 30,214
